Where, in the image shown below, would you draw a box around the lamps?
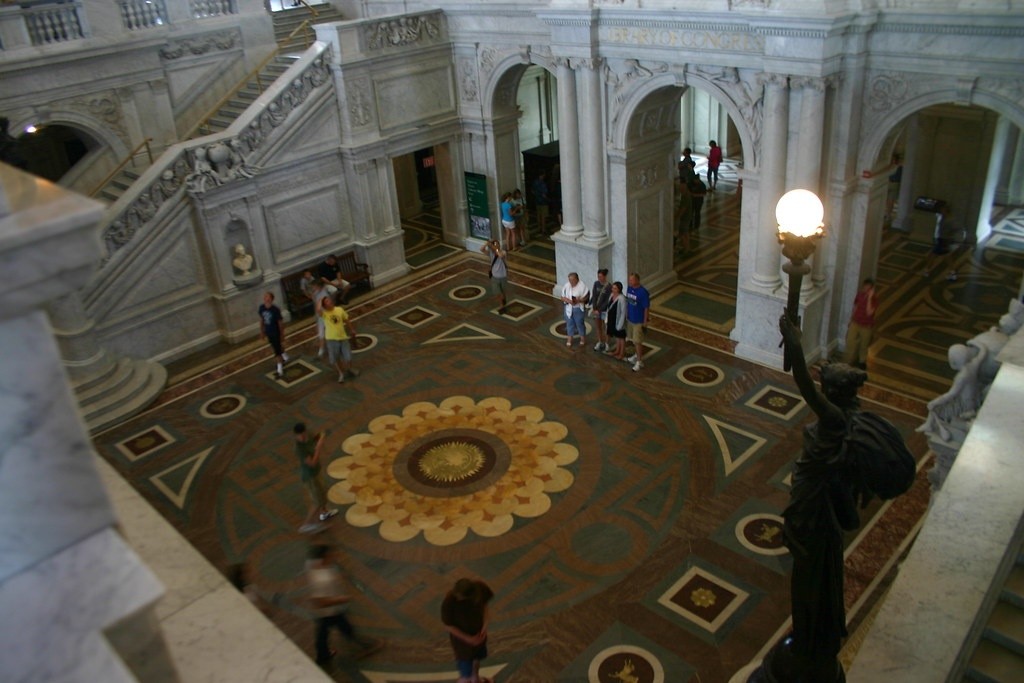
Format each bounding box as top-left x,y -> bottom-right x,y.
775,190 -> 826,243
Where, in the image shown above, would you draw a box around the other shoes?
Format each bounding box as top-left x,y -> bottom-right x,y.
566,341 -> 573,347
277,363 -> 284,374
611,351 -> 625,360
580,340 -> 587,347
348,368 -> 359,377
318,349 -> 326,357
281,353 -> 289,362
338,373 -> 345,383
340,297 -> 349,305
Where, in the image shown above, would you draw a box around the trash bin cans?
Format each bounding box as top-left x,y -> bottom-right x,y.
911,196 -> 951,246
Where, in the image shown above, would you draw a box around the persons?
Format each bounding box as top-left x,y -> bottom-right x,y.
304,543 -> 384,669
300,254 -> 361,383
293,422 -> 339,521
592,268 -> 650,372
706,140 -> 722,192
479,239 -> 509,315
500,189 -> 527,252
438,576 -> 494,683
257,292 -> 289,377
677,148 -> 706,256
561,272 -> 590,348
778,314 -> 870,658
843,278 -> 880,371
233,244 -> 253,276
193,139 -> 258,193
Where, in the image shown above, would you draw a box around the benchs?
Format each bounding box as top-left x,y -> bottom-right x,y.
280,252 -> 371,319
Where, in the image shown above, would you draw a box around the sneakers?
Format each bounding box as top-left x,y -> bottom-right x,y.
318,508 -> 339,521
301,524 -> 318,535
632,360 -> 644,371
605,343 -> 611,352
628,354 -> 637,363
594,342 -> 605,351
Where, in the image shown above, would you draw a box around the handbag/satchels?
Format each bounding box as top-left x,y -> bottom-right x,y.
488,271 -> 492,279
